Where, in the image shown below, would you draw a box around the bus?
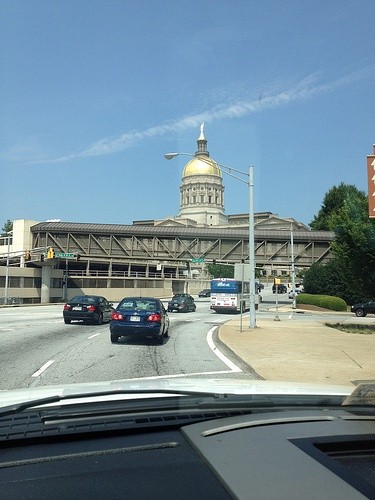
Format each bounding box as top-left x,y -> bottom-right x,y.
209,278 -> 264,313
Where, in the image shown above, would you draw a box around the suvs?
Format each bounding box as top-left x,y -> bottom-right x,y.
272,284 -> 287,294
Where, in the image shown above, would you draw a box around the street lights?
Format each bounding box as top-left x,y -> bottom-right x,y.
164,153 -> 257,328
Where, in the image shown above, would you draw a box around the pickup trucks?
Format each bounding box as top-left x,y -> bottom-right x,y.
288,279 -> 305,292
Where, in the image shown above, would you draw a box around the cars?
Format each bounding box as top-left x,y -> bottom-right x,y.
351,298 -> 375,317
63,295 -> 116,324
109,296 -> 170,344
168,293 -> 196,313
288,289 -> 301,299
198,289 -> 211,297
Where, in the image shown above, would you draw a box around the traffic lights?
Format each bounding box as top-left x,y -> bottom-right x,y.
213,259 -> 216,266
27,250 -> 30,260
40,254 -> 44,262
51,248 -> 55,258
186,260 -> 190,268
273,278 -> 280,285
